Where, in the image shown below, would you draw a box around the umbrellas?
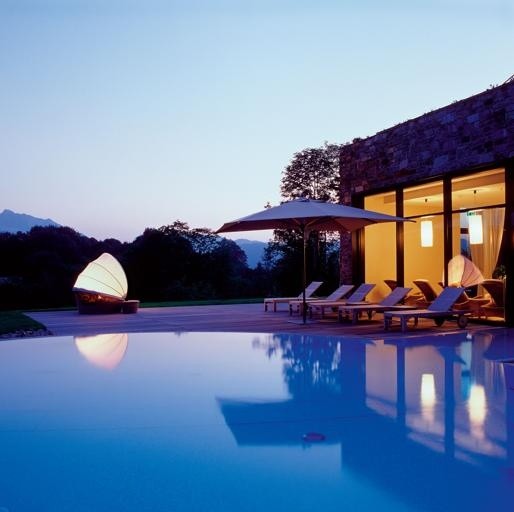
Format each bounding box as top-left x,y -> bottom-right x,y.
210,196 -> 416,325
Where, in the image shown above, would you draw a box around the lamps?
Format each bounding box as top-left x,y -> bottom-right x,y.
421,198 -> 433,248
467,189 -> 483,245
465,383 -> 490,447
419,373 -> 437,432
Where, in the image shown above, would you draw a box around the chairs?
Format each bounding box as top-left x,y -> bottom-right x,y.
264,279 -> 506,332
358,331 -> 514,364
